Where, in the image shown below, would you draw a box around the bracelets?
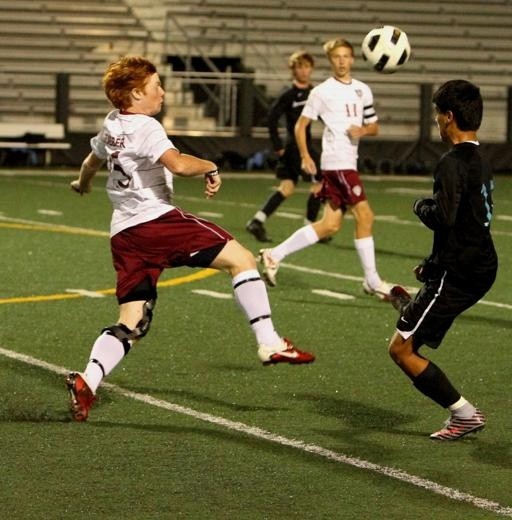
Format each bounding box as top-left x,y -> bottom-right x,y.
207,169 -> 219,177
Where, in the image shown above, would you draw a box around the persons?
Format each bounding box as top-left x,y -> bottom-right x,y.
246,50 -> 334,248
63,53 -> 317,421
388,80 -> 499,441
259,37 -> 406,302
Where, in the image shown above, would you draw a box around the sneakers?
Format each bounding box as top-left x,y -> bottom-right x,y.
430,407 -> 486,441
259,248 -> 279,286
363,281 -> 393,303
258,337 -> 315,367
66,370 -> 96,422
390,286 -> 411,312
246,216 -> 271,242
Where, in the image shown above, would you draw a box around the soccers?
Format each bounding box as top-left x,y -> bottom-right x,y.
363,24 -> 411,73
